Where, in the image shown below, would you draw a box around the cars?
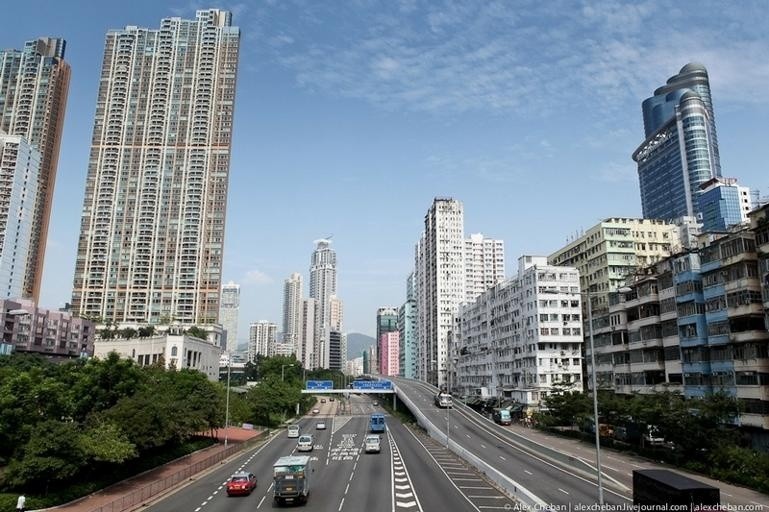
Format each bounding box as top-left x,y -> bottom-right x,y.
312,408 -> 321,414
226,469 -> 257,496
316,421 -> 327,430
329,397 -> 335,402
373,399 -> 379,406
320,399 -> 326,403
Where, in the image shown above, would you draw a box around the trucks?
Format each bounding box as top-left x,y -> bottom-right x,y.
365,434 -> 384,454
369,412 -> 385,433
435,391 -> 453,409
272,454 -> 314,506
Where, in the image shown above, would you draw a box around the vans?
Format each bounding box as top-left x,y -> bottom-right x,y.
297,434 -> 316,452
286,423 -> 301,438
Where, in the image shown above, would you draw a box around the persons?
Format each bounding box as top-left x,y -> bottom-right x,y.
13,489 -> 28,512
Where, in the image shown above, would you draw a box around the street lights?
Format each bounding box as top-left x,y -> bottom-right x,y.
281,363 -> 295,385
545,284 -> 633,512
224,348 -> 240,447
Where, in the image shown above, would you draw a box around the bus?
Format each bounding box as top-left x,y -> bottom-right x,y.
491,406 -> 512,427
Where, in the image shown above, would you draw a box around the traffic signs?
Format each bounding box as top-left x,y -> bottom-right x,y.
353,380 -> 392,390
305,380 -> 334,391
